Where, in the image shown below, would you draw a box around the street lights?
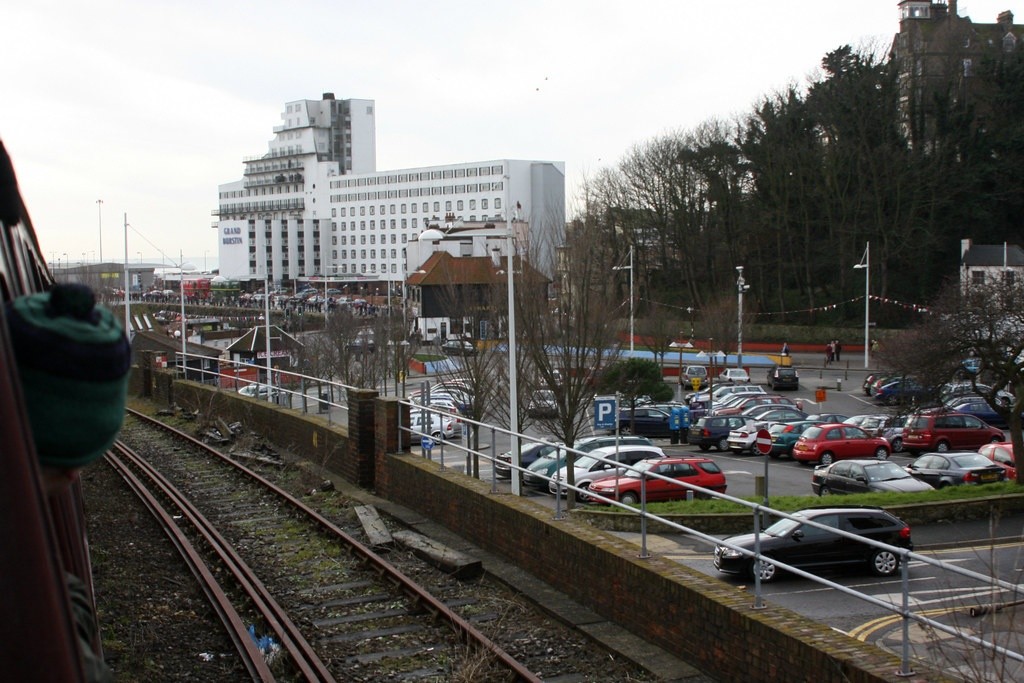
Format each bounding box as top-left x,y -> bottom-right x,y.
48,200 -> 210,277
495,246 -> 523,307
417,159 -> 519,497
854,242 -> 869,368
367,259 -> 427,326
669,338 -> 727,407
612,245 -> 634,359
325,253 -> 344,328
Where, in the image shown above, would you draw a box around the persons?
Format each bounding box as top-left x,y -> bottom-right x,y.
831,341 -> 836,360
826,343 -> 832,365
835,340 -> 841,361
783,342 -> 789,354
872,339 -> 879,353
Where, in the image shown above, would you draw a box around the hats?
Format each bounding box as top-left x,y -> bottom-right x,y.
6,282 -> 132,469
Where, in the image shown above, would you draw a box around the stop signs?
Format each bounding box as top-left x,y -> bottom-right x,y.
757,429 -> 773,454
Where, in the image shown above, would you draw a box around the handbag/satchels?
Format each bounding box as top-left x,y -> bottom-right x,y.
785,348 -> 789,353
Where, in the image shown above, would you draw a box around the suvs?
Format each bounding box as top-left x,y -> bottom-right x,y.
589,455 -> 727,507
714,504 -> 913,584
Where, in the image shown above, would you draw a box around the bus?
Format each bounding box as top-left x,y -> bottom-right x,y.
181,279 -> 241,301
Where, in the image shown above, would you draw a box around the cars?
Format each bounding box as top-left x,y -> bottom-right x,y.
253,286 -> 368,307
408,340 -> 683,504
978,442 -> 1016,481
902,414 -> 1006,457
812,460 -> 936,497
143,290 -> 173,298
901,450 -> 1006,491
680,356 -> 1014,468
